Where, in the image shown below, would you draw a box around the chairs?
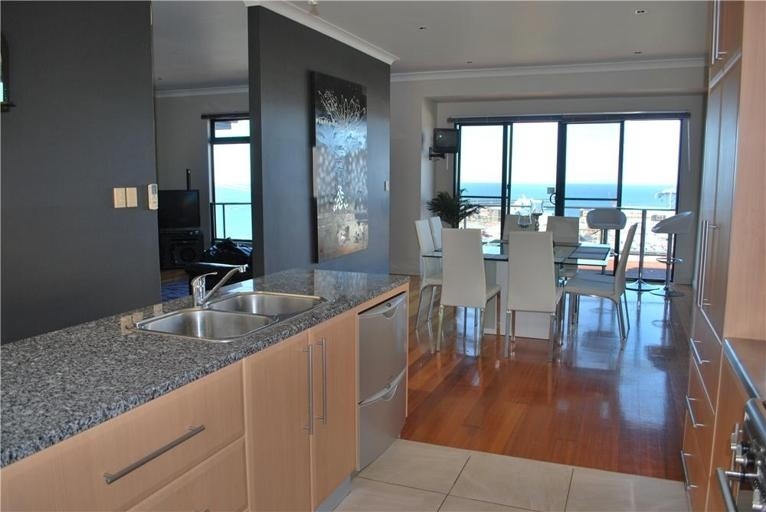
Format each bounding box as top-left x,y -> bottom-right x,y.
436,228 -> 500,359
548,216 -> 579,244
430,217 -> 478,325
652,211 -> 693,296
502,215 -> 535,241
414,220 -> 442,329
587,209 -> 624,274
565,224 -> 638,350
504,232 -> 564,363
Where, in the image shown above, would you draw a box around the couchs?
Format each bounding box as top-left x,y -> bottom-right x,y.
185,248 -> 252,295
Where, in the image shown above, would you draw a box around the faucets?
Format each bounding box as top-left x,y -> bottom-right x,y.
190,264 -> 248,306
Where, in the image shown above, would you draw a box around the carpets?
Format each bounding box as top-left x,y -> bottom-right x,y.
161,280 -> 189,301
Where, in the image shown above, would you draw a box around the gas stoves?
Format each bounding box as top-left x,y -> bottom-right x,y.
729,396 -> 766,512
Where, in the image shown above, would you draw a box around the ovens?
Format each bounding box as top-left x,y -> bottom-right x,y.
716,453 -> 766,512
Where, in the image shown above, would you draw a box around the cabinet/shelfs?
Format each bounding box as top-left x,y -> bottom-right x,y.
241,311 -> 356,512
159,230 -> 204,269
0,359 -> 249,512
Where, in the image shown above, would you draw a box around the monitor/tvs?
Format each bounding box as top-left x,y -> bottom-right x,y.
433,128 -> 459,153
158,190 -> 201,229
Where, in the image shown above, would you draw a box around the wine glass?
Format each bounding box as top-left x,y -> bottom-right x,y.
515,199 -> 545,232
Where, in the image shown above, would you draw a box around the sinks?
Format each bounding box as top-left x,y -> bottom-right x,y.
205,292 -> 328,315
136,308 -> 278,342
